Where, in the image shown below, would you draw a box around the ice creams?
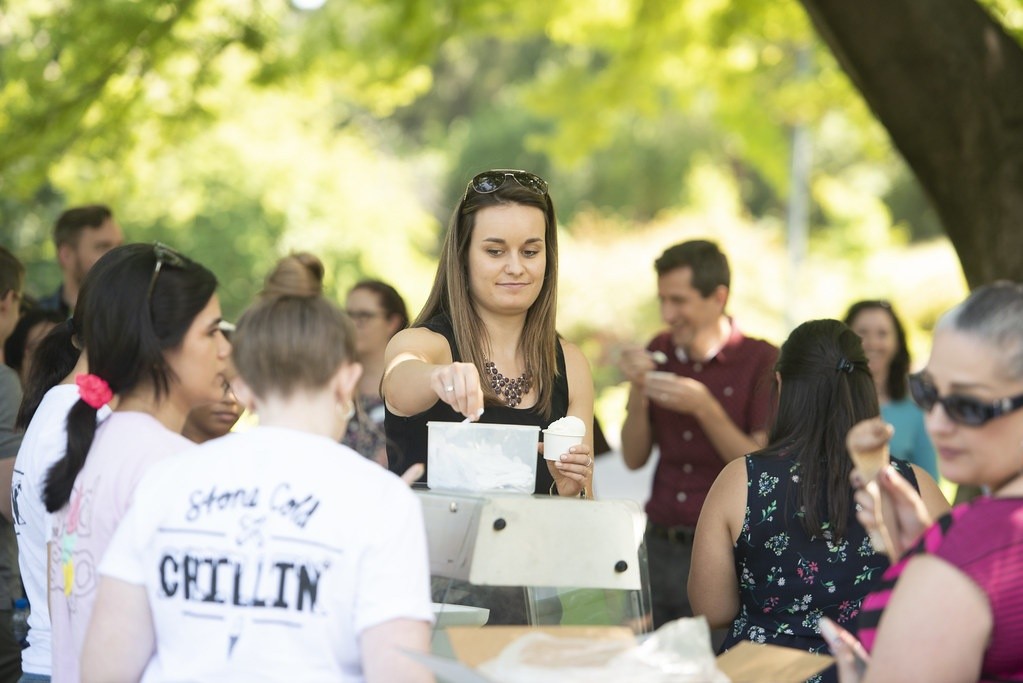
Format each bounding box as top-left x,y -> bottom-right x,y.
846,418 -> 894,492
546,415 -> 586,437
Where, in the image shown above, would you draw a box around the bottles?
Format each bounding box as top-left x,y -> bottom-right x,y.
13,597 -> 31,648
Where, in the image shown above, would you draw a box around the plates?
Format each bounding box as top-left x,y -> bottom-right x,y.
432,603 -> 491,628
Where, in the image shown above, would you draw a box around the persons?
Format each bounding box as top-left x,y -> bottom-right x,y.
617,238 -> 782,638
849,282 -> 1022,682
378,167 -> 597,626
0,204 -> 434,680
841,299 -> 939,481
688,319 -> 956,682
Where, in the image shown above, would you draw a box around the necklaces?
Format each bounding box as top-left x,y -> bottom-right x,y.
481,349 -> 537,407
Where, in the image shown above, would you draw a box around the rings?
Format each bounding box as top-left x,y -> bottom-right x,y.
445,386 -> 454,392
587,455 -> 594,469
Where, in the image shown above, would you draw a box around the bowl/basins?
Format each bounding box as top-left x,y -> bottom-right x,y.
542,430 -> 584,461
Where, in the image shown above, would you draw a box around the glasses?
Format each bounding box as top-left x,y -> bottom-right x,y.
461,168 -> 548,207
147,240 -> 181,299
908,370 -> 1023,427
